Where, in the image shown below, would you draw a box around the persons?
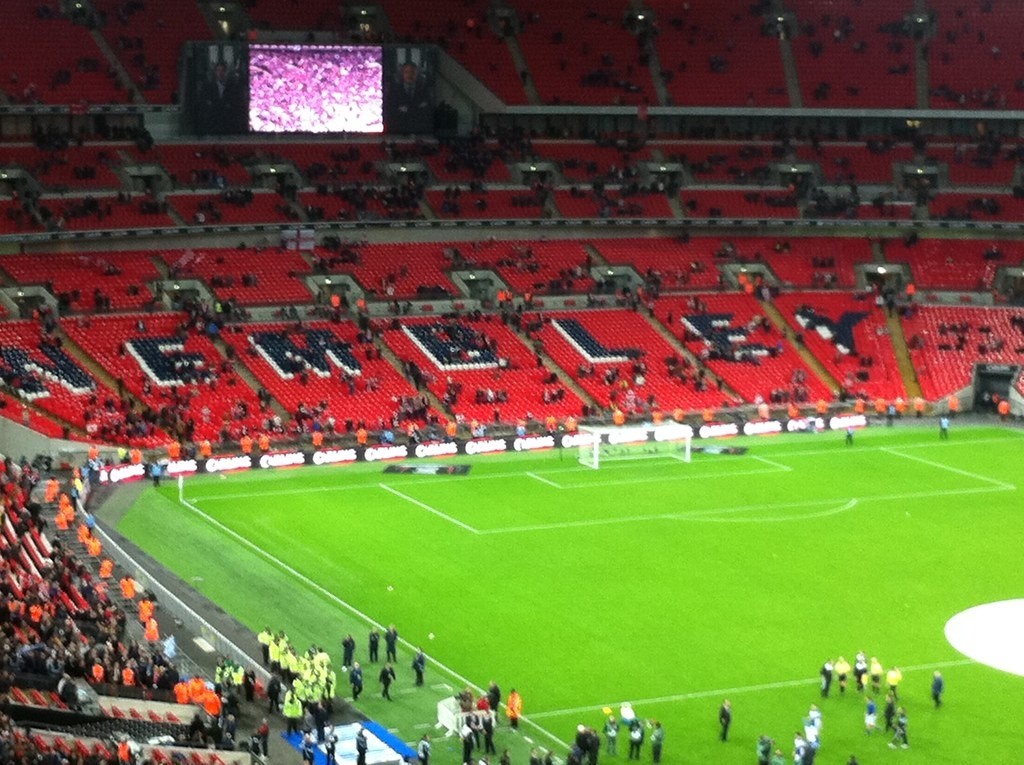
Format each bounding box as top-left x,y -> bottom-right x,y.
173,623 -> 944,765
0,446 -> 161,765
0,1 -> 1024,445
